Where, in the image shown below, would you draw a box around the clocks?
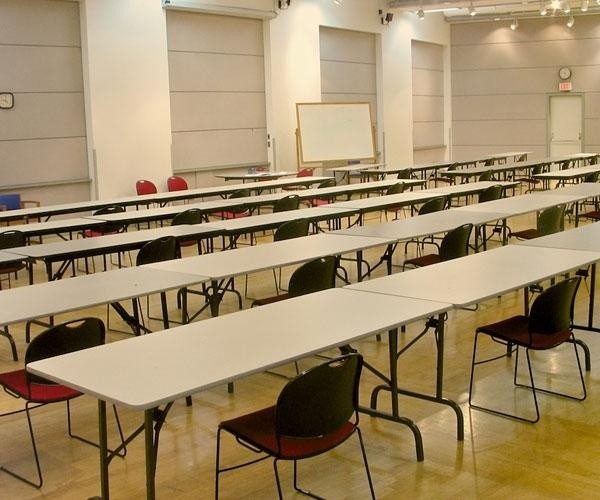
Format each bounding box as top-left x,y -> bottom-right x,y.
559,66 -> 572,81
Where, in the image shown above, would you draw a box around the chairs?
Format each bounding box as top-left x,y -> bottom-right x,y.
1,147 -> 599,488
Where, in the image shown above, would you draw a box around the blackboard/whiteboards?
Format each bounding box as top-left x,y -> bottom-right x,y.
296,101 -> 377,163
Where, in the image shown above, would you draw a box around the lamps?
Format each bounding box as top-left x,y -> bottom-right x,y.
417,0 -> 600,29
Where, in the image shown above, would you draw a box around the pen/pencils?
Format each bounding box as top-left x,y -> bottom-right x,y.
262,174 -> 271,175
